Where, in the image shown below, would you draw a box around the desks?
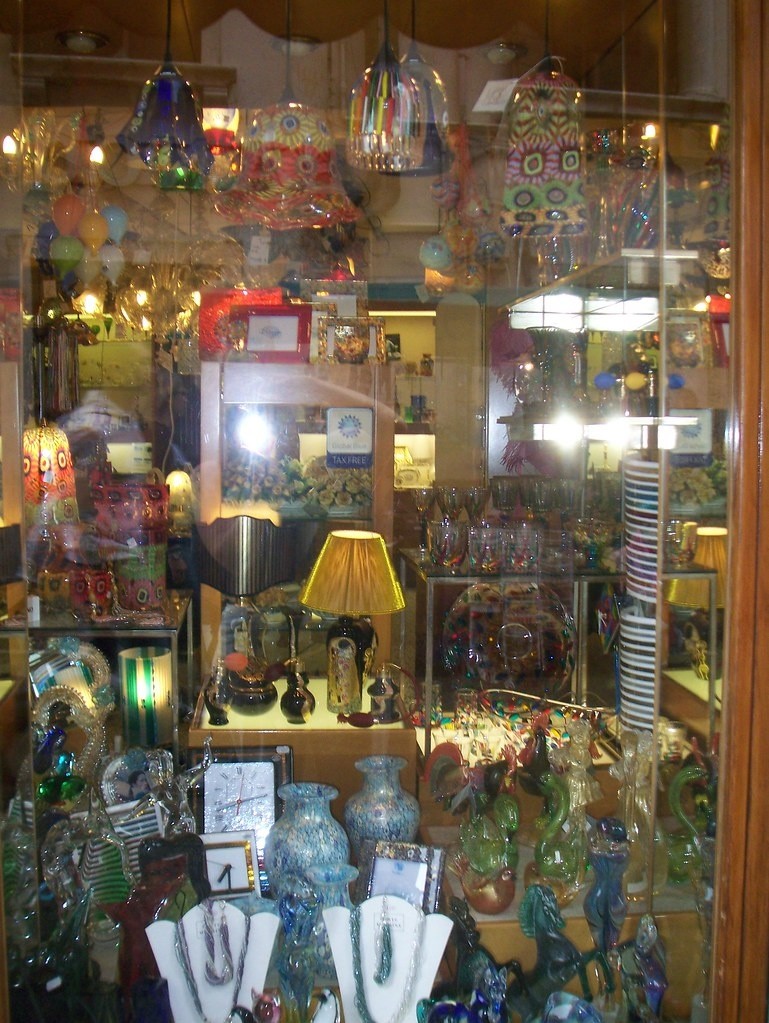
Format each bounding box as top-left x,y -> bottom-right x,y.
1,587 -> 203,775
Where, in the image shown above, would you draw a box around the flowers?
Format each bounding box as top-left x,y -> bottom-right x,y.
221,444 -> 307,505
304,455 -> 374,511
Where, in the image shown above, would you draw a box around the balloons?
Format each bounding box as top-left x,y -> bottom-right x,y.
34,194 -> 130,289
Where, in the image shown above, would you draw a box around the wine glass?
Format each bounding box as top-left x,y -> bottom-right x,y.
410,488 -> 438,553
434,486 -> 466,521
463,487 -> 492,523
518,477 -> 580,568
489,476 -> 519,522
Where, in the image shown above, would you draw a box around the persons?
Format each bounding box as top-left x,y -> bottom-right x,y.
109,731 -> 213,921
552,719 -> 603,886
609,730 -> 665,881
128,770 -> 150,801
29,812 -> 140,972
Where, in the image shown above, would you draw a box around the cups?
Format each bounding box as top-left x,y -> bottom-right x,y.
405,406 -> 413,423
422,409 -> 434,422
502,528 -> 540,574
619,602 -> 656,736
411,395 -> 426,422
623,451 -> 664,603
572,519 -> 610,569
118,645 -> 175,748
468,523 -> 505,575
114,541 -> 168,611
69,568 -> 113,621
36,571 -> 69,609
427,521 -> 468,568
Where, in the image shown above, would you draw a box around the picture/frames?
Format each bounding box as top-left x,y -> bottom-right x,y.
311,302 -> 337,363
369,840 -> 445,916
229,305 -> 313,364
318,316 -> 386,366
300,278 -> 369,319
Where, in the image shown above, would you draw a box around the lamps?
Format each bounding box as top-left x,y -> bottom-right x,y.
0,1 -> 768,1023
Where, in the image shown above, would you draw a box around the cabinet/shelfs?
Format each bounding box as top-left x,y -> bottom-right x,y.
185,357 -> 418,840
399,549 -> 719,1023
369,309 -> 440,590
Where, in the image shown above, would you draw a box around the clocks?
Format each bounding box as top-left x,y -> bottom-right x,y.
196,829 -> 262,903
187,744 -> 295,890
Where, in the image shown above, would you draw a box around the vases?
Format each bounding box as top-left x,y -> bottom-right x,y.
305,862 -> 360,982
343,754 -> 420,866
421,353 -> 436,378
264,782 -> 350,902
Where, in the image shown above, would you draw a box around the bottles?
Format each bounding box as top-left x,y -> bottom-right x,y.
304,863 -> 360,980
343,753 -> 420,866
394,384 -> 401,419
420,353 -> 434,376
264,782 -> 351,900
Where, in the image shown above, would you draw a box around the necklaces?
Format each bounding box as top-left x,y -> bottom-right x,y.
349,892 -> 427,1023
372,896 -> 393,986
176,897 -> 251,1022
201,897 -> 234,986
